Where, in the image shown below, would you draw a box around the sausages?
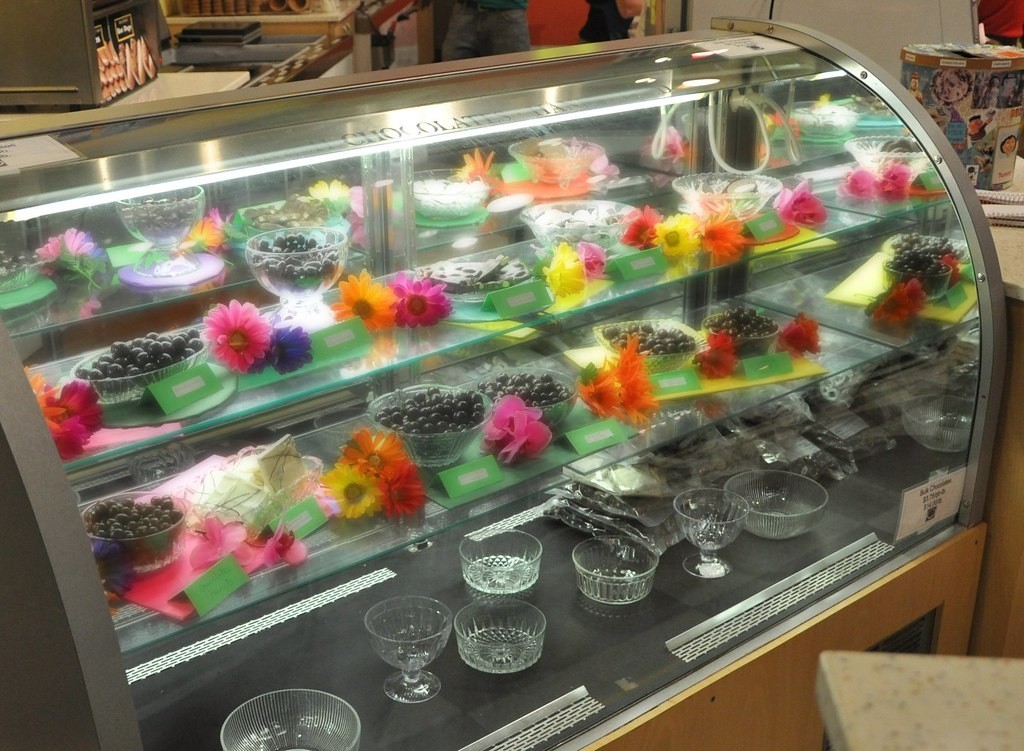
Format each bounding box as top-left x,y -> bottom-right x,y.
97,41 -> 127,102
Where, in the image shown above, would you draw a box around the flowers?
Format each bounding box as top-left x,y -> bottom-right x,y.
941,254 -> 961,288
836,167 -> 878,198
874,165 -> 912,202
864,278 -> 926,324
23,148 -> 827,574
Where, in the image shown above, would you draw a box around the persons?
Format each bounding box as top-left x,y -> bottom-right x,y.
578,0 -> 644,44
440,0 -> 531,60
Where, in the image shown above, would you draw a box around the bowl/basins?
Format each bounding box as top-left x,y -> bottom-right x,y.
81,491 -> 187,575
882,261 -> 953,301
592,318 -> 710,372
458,529 -> 543,594
365,384 -> 494,467
220,689 -> 361,751
474,367 -> 576,429
0,249 -> 45,293
572,535 -> 660,605
520,170 -> 783,253
723,469 -> 829,542
454,597 -> 547,674
412,136 -> 606,218
901,393 -> 975,453
784,101 -> 930,187
70,336 -> 207,402
701,312 -> 781,362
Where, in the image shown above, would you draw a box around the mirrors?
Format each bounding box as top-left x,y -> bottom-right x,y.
742,71 -> 983,361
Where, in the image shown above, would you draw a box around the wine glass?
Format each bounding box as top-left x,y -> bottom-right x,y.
364,595 -> 452,704
246,226 -> 349,337
115,185 -> 205,282
673,488 -> 749,578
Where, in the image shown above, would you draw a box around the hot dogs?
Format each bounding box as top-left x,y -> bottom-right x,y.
117,35 -> 157,90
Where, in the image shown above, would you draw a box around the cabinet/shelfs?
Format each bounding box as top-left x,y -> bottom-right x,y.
0,18 -> 1009,751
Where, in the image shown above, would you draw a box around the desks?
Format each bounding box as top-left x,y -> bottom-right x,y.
814,651 -> 1024,751
966,223 -> 1024,659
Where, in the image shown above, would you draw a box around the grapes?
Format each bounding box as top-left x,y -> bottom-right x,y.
885,232 -> 962,275
84,496 -> 183,566
602,304 -> 778,357
77,328 -> 204,394
260,232 -> 341,293
127,200 -> 198,232
374,370 -> 570,459
